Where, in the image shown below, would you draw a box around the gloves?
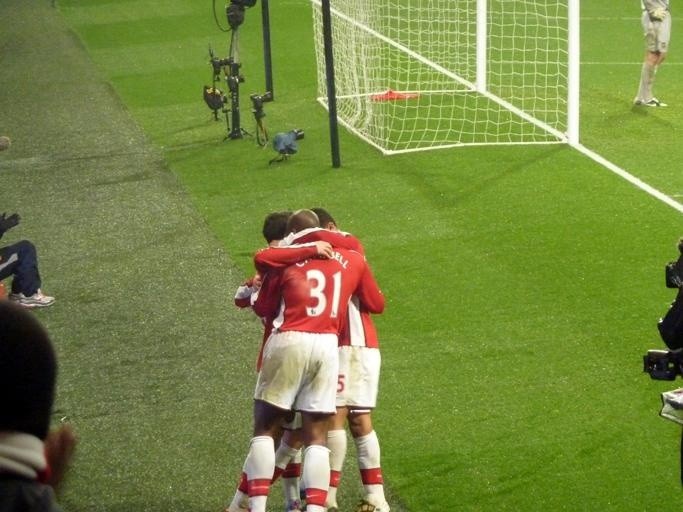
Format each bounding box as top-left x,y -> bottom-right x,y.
1,212 -> 21,231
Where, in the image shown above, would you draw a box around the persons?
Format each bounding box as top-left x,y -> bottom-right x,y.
632,1 -> 673,109
0,296 -> 78,510
226,206 -> 391,512
0,210 -> 54,309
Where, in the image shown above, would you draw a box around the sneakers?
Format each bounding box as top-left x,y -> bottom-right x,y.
7,292 -> 23,304
641,96 -> 667,107
632,97 -> 644,105
18,288 -> 56,307
